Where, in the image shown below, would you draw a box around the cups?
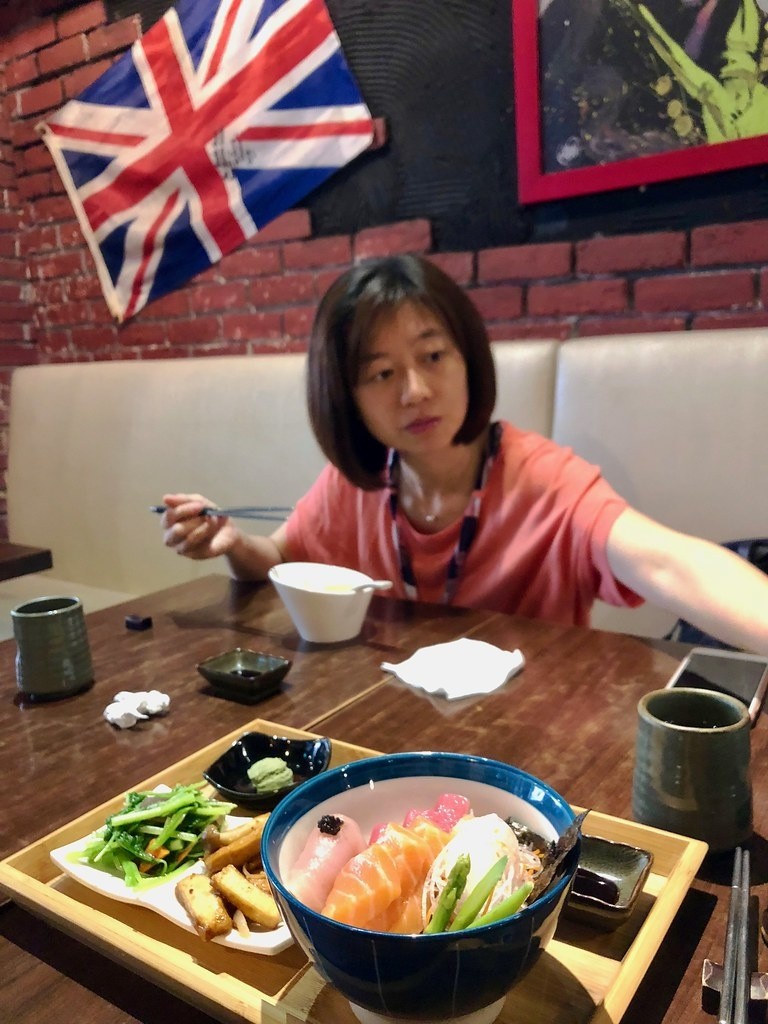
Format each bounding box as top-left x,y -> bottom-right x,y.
10,593 -> 94,700
630,687 -> 753,862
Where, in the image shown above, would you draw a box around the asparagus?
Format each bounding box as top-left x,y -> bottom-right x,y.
421,854 -> 534,934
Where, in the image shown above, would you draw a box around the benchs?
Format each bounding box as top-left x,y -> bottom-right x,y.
0,327 -> 768,642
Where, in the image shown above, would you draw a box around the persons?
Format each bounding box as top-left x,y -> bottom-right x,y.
160,256 -> 768,655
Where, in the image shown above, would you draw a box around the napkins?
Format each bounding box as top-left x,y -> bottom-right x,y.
380,639 -> 524,702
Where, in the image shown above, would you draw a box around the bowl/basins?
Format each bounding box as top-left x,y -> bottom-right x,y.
262,750 -> 583,1024
203,731 -> 331,812
194,645 -> 292,701
268,562 -> 377,643
569,833 -> 656,924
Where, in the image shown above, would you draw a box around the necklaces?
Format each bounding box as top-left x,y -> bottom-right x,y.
401,466 -> 475,522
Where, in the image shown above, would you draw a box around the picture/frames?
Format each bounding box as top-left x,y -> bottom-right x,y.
511,0 -> 768,207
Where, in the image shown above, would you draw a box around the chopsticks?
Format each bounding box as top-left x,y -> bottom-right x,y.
150,503 -> 295,522
719,848 -> 753,1024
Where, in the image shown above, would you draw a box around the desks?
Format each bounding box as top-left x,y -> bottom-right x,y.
0,571 -> 767,1024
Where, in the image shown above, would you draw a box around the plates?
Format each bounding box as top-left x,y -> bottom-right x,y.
49,785 -> 308,957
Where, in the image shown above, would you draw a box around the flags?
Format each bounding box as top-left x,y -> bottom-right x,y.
35,0 -> 376,323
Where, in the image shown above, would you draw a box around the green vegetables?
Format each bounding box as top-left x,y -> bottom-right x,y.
64,779 -> 236,892
